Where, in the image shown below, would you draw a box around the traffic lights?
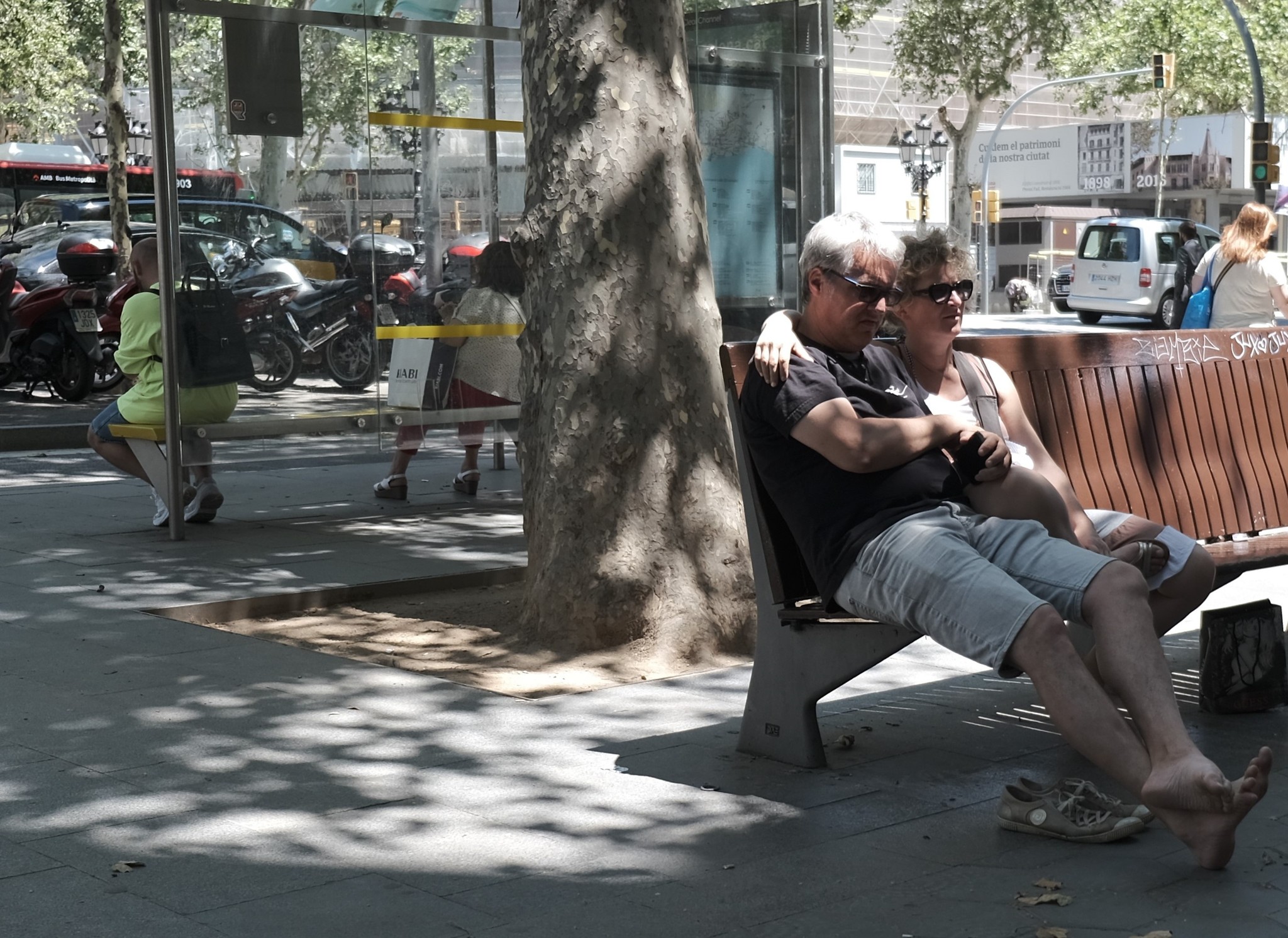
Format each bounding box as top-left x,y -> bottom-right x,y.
344,172 -> 359,202
1154,54 -> 1166,89
975,201 -> 982,224
1252,121 -> 1280,185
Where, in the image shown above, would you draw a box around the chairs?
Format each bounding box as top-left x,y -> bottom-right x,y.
1108,241 -> 1123,258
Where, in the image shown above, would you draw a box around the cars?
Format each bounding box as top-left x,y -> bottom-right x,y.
0,222 -> 328,292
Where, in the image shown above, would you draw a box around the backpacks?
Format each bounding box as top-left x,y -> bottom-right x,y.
146,261 -> 256,388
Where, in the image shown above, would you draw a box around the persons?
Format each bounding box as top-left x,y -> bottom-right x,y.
370,242 -> 527,501
1191,202 -> 1288,330
1174,220 -> 1206,330
738,210 -> 1270,872
751,221 -> 1216,692
86,237 -> 238,526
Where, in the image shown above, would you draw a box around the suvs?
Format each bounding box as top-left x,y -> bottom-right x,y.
1067,215 -> 1221,330
1046,237 -> 1172,313
9,194 -> 350,279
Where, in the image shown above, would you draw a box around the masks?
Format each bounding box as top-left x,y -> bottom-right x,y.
1179,237 -> 1185,246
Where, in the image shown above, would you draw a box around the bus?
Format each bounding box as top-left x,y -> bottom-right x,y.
1,142 -> 243,238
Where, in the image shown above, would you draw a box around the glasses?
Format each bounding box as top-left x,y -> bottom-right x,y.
824,268 -> 905,306
912,279 -> 974,304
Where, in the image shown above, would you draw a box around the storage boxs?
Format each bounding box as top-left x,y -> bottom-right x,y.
343,231 -> 417,280
443,226 -> 510,286
57,229 -> 120,283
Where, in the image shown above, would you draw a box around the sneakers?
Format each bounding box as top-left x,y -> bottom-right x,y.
184,475 -> 225,523
996,775 -> 1154,843
149,479 -> 197,526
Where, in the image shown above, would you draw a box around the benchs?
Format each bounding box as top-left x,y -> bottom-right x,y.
107,404 -> 521,543
717,326 -> 1288,770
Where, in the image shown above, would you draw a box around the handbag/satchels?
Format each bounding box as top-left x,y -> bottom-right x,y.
386,337 -> 459,411
1180,284 -> 1212,329
1196,597 -> 1288,713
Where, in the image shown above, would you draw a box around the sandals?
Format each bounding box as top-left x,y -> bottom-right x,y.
1111,539 -> 1169,579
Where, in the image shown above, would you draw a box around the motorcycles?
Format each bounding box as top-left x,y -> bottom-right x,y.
0,213 -> 303,402
213,213 -> 506,390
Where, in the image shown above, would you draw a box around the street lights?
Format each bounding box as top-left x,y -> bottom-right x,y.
402,70 -> 420,279
895,114 -> 949,240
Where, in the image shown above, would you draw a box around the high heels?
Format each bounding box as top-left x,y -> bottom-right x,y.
452,469 -> 481,495
373,473 -> 408,500
